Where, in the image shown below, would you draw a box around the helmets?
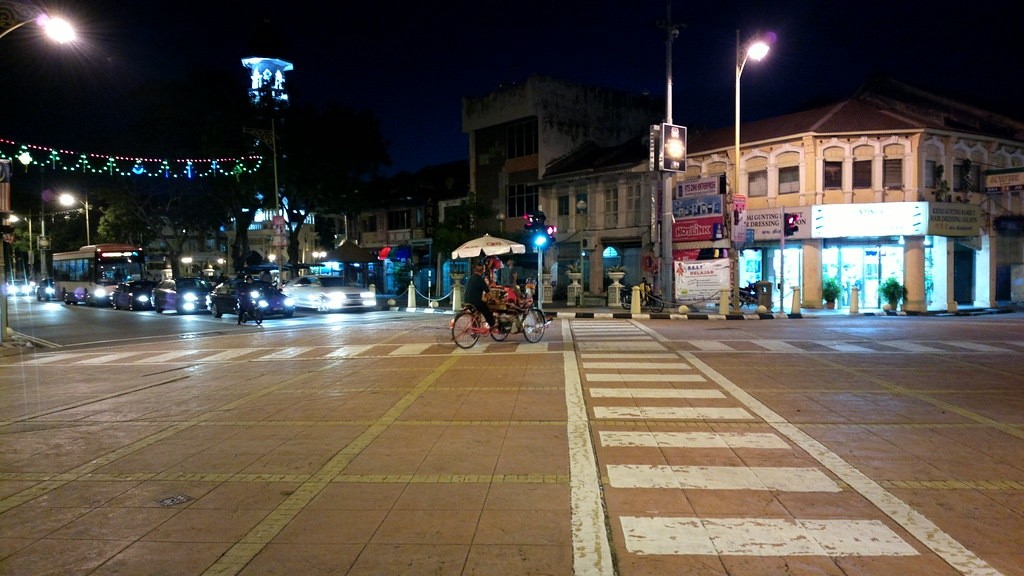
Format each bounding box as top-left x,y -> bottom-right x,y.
246,275 -> 254,281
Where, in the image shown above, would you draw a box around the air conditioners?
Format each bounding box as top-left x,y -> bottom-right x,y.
583,236 -> 596,250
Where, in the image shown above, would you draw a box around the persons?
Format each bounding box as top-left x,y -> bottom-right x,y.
114,268 -> 124,280
260,270 -> 272,287
463,256 -> 536,330
734,204 -> 739,225
237,275 -> 254,325
641,277 -> 647,284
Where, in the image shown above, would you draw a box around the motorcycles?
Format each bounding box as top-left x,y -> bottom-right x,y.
232,291 -> 263,324
729,280 -> 774,310
620,277 -> 665,313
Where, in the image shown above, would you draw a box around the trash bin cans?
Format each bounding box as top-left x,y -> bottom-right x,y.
756,281 -> 773,311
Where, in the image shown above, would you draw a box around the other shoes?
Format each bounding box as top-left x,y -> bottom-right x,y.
512,329 -> 524,334
490,322 -> 499,329
470,331 -> 480,336
236,321 -> 241,325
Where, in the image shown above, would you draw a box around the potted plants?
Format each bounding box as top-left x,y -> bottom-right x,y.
449,270 -> 466,304
542,265 -> 553,303
606,265 -> 626,306
878,276 -> 903,310
565,259 -> 581,305
822,278 -> 841,309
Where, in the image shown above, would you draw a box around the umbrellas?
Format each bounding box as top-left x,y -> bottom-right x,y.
452,233 -> 526,260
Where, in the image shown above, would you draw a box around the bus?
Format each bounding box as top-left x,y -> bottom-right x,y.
52,244 -> 148,307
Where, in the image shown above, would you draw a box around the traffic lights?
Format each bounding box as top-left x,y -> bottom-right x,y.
546,225 -> 558,247
524,213 -> 537,230
784,213 -> 799,237
535,233 -> 548,248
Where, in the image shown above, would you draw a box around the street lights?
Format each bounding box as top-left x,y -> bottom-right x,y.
241,57 -> 296,271
734,28 -> 771,317
59,193 -> 90,246
661,29 -> 680,304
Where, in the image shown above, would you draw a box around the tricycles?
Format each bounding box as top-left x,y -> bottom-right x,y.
449,284 -> 547,349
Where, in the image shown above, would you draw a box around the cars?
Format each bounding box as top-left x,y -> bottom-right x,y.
109,278 -> 296,319
280,275 -> 377,312
7,278 -> 55,302
